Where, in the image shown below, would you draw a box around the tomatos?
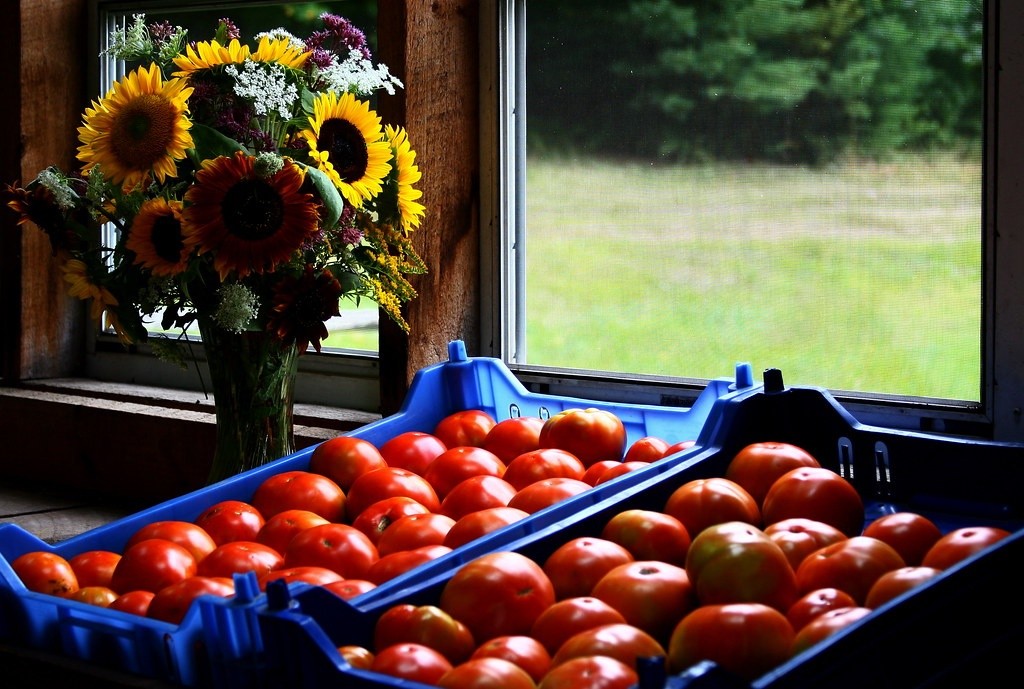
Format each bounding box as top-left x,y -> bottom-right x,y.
347,434 -> 1009,689
11,406 -> 697,624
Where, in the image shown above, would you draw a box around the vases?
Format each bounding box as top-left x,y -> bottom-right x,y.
199,312 -> 300,484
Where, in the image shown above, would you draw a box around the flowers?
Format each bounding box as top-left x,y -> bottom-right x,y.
2,2 -> 429,376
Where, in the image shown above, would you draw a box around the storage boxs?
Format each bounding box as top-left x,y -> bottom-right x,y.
0,338 -> 1024,689
